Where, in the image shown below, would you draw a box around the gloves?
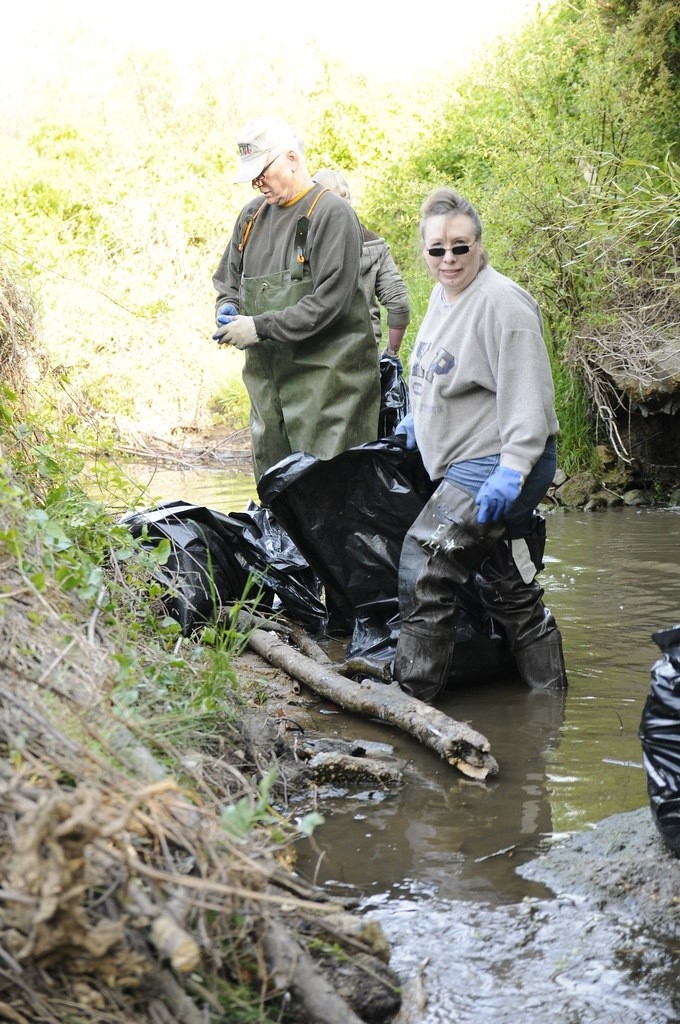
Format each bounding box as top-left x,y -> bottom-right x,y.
211,301 -> 259,349
395,413 -> 418,451
473,464 -> 524,526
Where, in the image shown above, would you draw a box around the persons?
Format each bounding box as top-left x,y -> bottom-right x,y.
389,191 -> 568,701
211,128 -> 381,640
313,171 -> 410,376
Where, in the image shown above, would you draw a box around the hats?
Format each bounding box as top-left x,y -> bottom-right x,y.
227,115 -> 297,185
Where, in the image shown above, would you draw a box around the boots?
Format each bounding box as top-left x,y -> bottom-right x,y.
348,627 -> 451,701
498,629 -> 569,693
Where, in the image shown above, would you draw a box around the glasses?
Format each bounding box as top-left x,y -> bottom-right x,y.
424,238 -> 481,258
